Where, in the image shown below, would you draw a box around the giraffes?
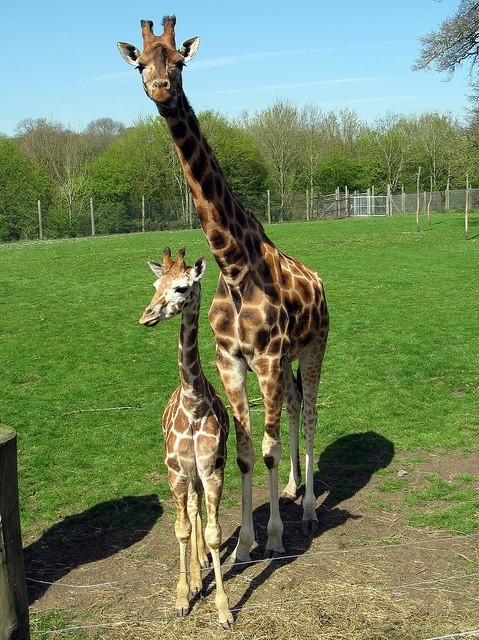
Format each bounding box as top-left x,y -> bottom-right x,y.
136,245 -> 237,632
117,14 -> 330,572
